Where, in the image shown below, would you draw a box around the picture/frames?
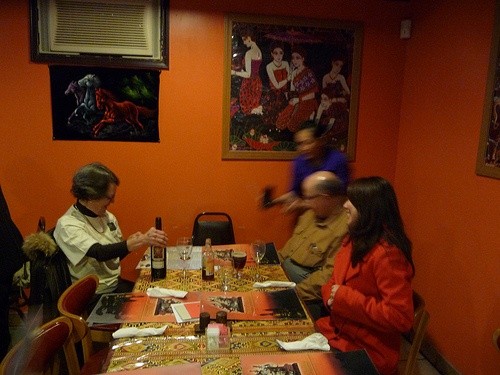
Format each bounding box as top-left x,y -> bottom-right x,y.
475,7 -> 500,179
221,12 -> 364,161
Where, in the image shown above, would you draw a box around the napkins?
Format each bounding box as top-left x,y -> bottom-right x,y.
277,332 -> 330,352
253,281 -> 296,288
112,324 -> 168,338
147,287 -> 187,298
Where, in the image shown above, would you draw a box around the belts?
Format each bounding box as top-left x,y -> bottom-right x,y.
289,258 -> 318,270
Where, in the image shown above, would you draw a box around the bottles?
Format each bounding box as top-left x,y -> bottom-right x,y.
201,238 -> 215,280
151,216 -> 167,279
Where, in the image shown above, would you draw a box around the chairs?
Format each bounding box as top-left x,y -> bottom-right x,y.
56,274 -> 121,364
405,288 -> 430,375
0,316 -> 74,375
192,212 -> 235,246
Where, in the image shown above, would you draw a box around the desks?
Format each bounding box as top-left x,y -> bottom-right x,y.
100,243 -> 336,375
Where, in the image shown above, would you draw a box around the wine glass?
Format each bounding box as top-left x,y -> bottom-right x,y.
220,257 -> 235,292
175,236 -> 193,277
230,251 -> 247,278
251,240 -> 267,279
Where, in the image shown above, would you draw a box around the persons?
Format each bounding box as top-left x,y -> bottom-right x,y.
276,171 -> 350,322
0,187 -> 29,361
254,120 -> 351,230
53,161 -> 168,310
314,176 -> 415,375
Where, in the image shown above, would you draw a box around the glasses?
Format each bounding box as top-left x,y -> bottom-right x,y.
104,194 -> 115,203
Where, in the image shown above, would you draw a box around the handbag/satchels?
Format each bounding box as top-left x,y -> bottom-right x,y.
10,261 -> 30,294
